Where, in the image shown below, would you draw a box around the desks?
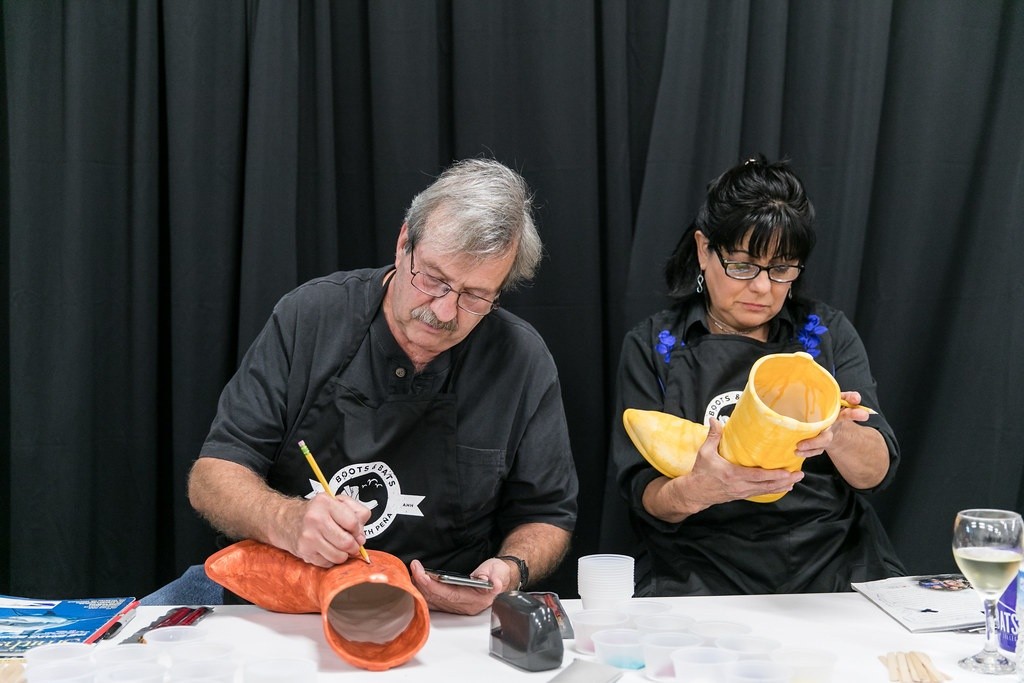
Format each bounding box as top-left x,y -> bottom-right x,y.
87,592 -> 1024,683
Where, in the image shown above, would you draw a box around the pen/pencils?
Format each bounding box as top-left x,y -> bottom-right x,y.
298,439 -> 371,566
544,593 -> 562,623
841,399 -> 879,415
137,606 -> 209,644
101,609 -> 137,640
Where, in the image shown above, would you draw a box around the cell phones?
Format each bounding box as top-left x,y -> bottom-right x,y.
423,569 -> 494,589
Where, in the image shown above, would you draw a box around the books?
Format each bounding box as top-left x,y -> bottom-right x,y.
851,574 -> 997,634
0,595 -> 141,658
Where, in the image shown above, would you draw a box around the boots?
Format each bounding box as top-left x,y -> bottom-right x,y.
620,351 -> 841,502
203,537 -> 431,670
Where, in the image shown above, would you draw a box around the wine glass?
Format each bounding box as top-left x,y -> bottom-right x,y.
952,509 -> 1024,675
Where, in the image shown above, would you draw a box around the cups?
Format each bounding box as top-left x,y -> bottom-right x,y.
22,626 -> 316,683
571,554 -> 834,683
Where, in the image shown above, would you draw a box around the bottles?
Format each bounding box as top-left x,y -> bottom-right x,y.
1015,562 -> 1024,683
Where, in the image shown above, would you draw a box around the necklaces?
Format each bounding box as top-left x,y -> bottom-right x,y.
706,301 -> 761,334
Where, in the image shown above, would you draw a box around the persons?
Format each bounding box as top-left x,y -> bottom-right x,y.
931,577 -> 949,590
615,149 -> 906,598
136,158 -> 578,616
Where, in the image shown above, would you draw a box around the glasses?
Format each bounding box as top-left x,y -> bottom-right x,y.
409,239 -> 502,316
711,244 -> 805,283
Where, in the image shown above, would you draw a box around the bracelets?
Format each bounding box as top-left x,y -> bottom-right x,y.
494,556 -> 529,591
